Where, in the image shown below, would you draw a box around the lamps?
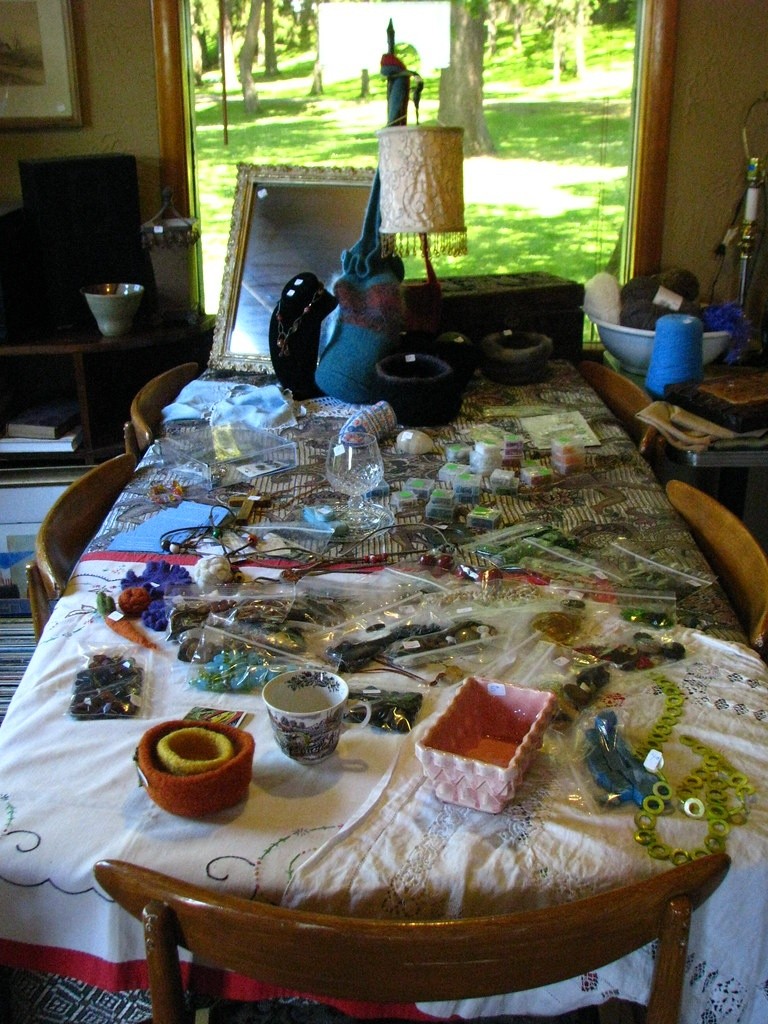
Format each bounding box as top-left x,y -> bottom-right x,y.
376,82 -> 468,303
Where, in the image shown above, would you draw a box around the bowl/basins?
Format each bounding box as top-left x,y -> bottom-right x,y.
588,313 -> 732,376
413,676 -> 555,814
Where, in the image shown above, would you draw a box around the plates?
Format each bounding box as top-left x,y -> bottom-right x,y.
298,502 -> 394,543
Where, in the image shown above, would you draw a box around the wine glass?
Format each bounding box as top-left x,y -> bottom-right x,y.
326,431 -> 384,528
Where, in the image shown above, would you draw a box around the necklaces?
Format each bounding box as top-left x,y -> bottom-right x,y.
276,282 -> 327,358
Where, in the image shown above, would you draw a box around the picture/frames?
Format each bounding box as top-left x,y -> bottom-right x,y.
0,0 -> 83,130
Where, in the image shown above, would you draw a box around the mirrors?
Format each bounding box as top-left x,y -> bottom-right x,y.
207,160 -> 391,374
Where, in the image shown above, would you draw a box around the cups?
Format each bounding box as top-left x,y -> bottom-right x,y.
260,670 -> 371,766
84,283 -> 145,336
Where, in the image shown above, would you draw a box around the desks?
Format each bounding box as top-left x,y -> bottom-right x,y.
1,351 -> 767,1024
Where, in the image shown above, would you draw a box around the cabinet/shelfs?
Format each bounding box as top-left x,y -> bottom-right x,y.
0,311 -> 215,490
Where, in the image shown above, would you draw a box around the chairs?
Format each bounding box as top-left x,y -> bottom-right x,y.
665,477 -> 768,655
28,449 -> 138,638
124,361 -> 198,459
97,852 -> 731,1021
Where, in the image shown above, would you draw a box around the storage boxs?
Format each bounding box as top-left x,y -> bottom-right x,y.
415,675 -> 554,814
406,272 -> 585,361
